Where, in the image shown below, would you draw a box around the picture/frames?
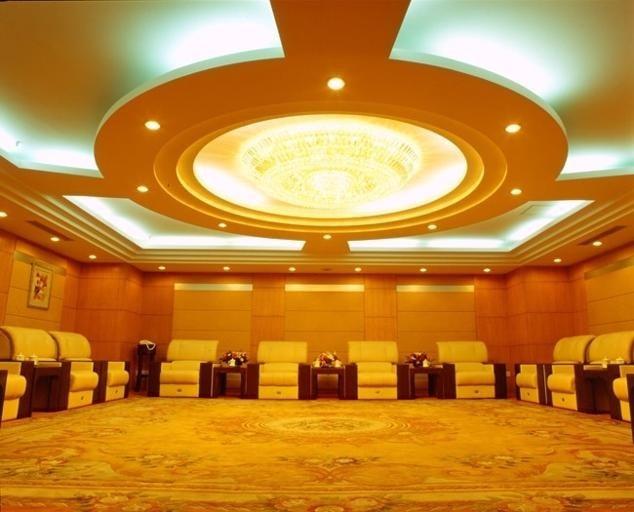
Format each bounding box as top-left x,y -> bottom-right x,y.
23,261 -> 55,312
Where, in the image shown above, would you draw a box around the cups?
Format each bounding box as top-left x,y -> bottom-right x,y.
228,359 -> 235,366
16,355 -> 24,361
29,356 -> 38,364
314,358 -> 320,367
334,360 -> 342,367
616,359 -> 625,365
422,359 -> 430,368
601,360 -> 610,368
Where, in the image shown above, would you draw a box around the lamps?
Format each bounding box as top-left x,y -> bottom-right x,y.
231,116 -> 429,212
